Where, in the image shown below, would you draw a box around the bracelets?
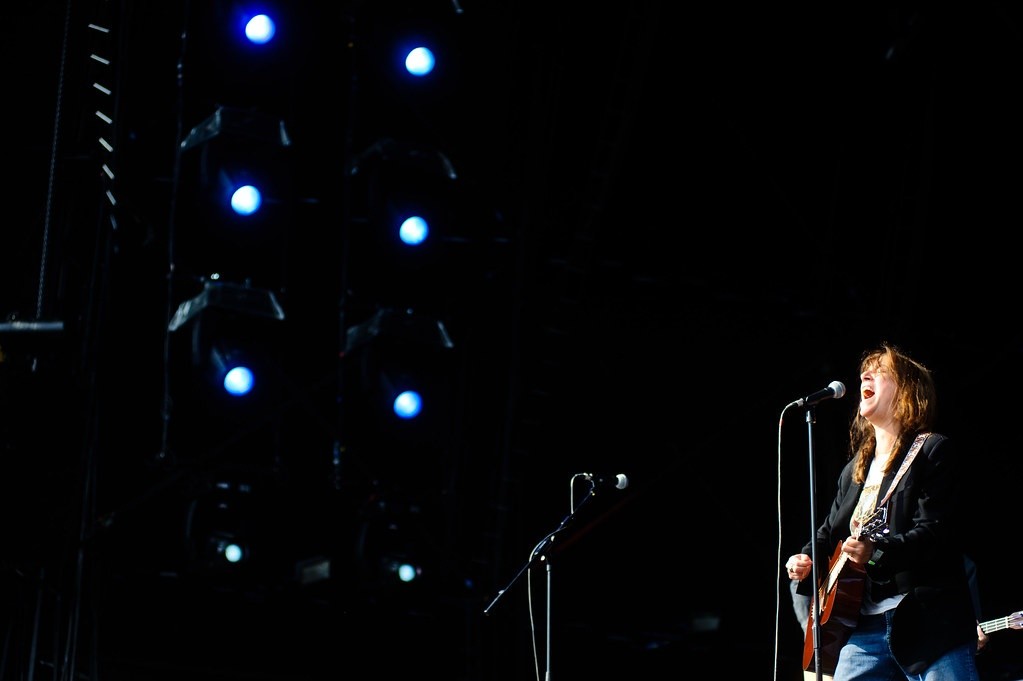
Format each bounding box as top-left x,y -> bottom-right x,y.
868,549 -> 884,565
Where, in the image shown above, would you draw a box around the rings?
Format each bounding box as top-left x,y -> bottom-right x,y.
789,565 -> 793,572
848,553 -> 853,557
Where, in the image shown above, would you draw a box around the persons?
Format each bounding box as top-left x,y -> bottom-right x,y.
785,346 -> 980,681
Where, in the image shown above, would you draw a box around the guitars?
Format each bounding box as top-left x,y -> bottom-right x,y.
802,506 -> 890,678
977,610 -> 1023,634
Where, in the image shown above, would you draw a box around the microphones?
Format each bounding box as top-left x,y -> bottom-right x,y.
785,380 -> 845,409
575,473 -> 628,489
